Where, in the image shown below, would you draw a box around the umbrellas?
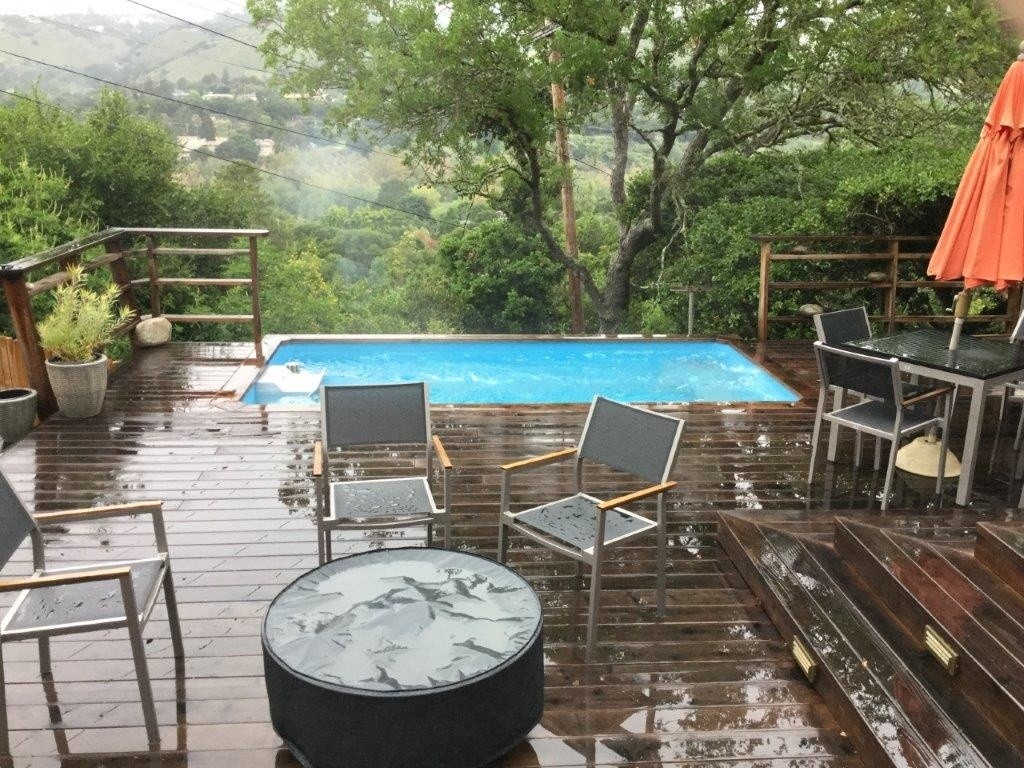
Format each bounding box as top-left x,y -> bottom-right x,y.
926,39 -> 1024,442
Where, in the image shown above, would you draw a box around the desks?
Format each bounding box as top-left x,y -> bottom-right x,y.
258,547 -> 547,768
834,325 -> 1023,508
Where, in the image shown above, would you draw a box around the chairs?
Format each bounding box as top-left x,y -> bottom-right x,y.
312,382 -> 458,567
494,395 -> 687,666
0,472 -> 188,747
805,339 -> 954,512
812,309 -> 920,471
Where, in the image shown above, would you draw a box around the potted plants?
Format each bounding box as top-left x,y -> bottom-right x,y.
32,262 -> 140,418
